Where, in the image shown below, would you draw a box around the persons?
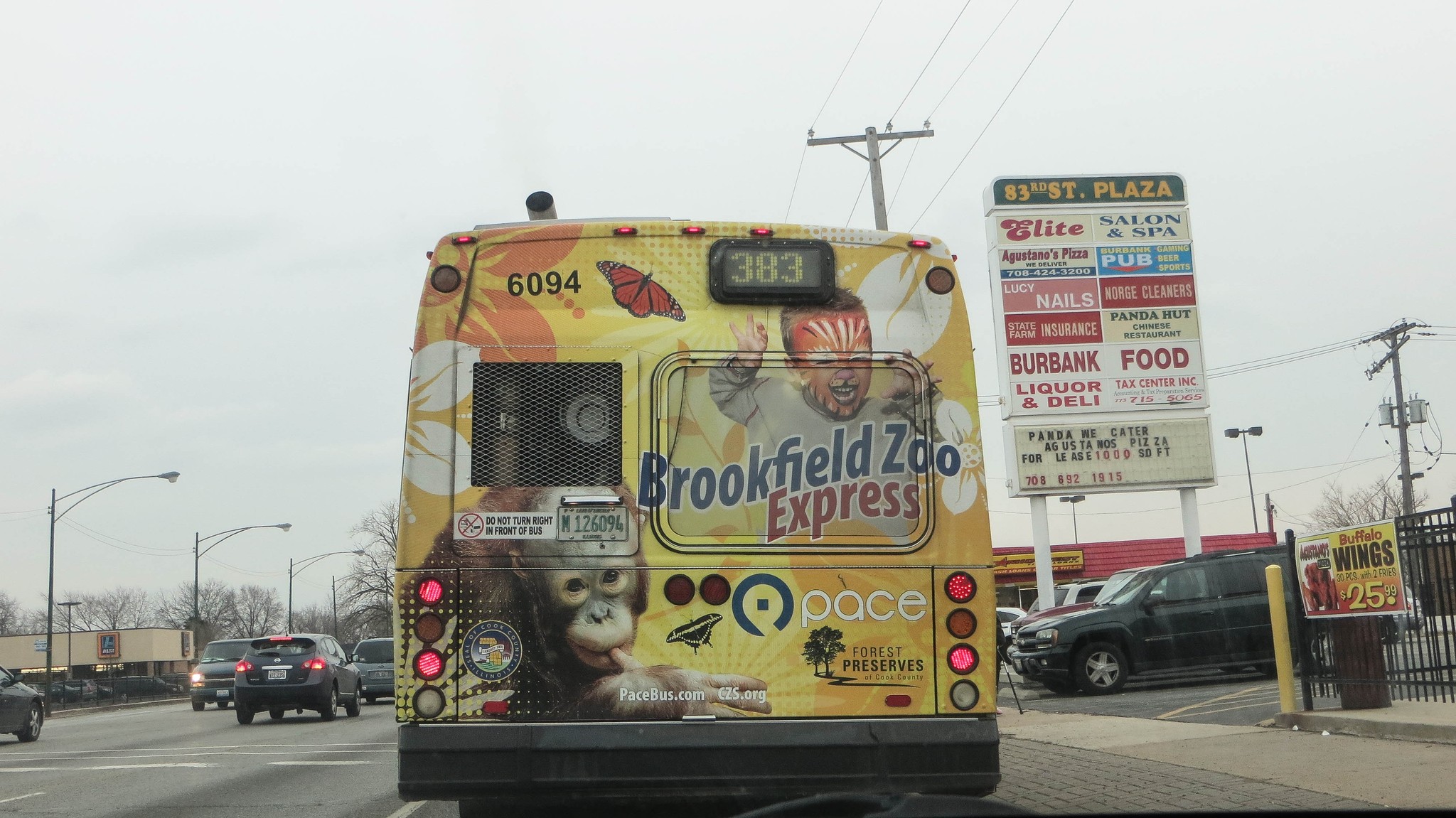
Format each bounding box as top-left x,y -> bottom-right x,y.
995,610 -> 1006,715
706,285 -> 945,491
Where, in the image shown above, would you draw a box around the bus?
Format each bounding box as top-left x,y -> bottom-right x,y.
390,191 -> 1003,818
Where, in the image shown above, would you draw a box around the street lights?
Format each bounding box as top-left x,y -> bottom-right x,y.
288,550 -> 365,634
44,471 -> 182,718
1222,425 -> 1263,533
1397,472 -> 1425,534
1059,495 -> 1086,543
332,568 -> 386,640
193,523 -> 293,667
57,601 -> 83,680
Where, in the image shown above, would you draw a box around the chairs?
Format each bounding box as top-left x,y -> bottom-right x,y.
279,646 -> 293,656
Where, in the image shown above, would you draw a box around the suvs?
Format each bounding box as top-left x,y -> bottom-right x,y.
190,638 -> 256,712
1005,544 -> 1395,697
350,635 -> 395,703
996,564 -> 1168,665
233,634 -> 363,725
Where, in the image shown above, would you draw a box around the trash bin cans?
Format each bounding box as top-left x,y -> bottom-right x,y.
1329,614 -> 1393,711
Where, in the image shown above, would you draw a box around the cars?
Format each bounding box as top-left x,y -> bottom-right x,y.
1393,584 -> 1424,631
24,670 -> 190,704
0,664 -> 44,742
